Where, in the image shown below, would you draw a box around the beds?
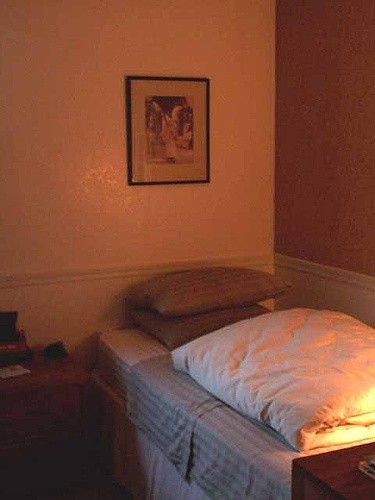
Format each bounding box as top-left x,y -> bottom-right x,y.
92,328 -> 375,500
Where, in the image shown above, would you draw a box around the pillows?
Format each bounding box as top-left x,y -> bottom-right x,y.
123,266 -> 289,319
123,303 -> 272,351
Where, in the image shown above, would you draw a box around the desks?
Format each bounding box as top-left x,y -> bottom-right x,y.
291,442 -> 375,500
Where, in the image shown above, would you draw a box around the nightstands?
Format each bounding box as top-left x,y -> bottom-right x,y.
0,353 -> 90,488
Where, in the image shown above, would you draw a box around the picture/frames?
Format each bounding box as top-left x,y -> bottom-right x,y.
124,74 -> 210,187
